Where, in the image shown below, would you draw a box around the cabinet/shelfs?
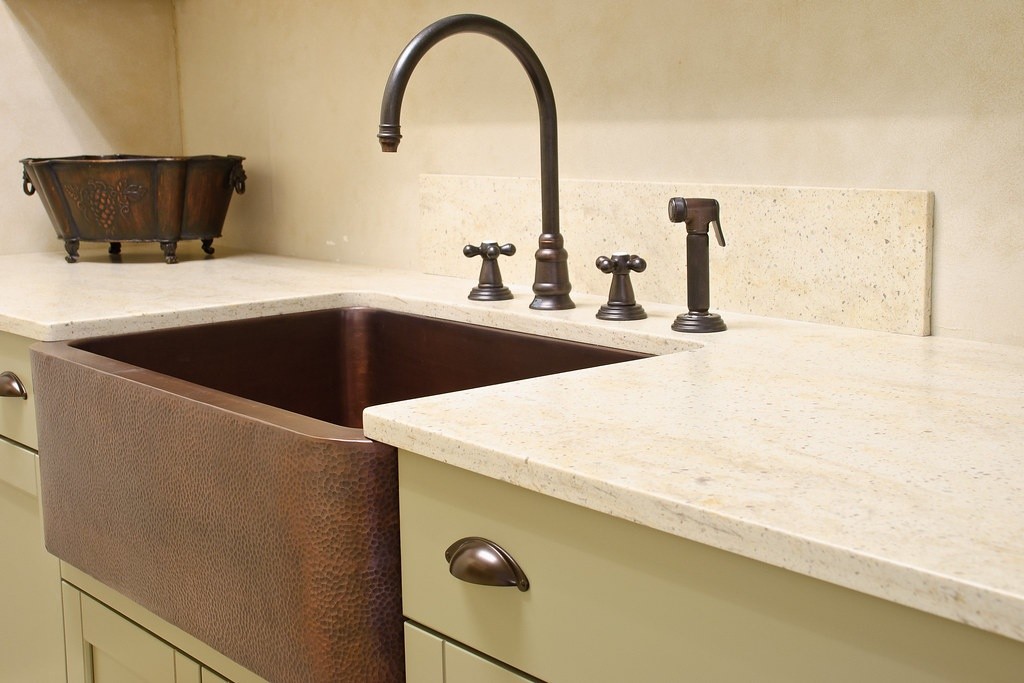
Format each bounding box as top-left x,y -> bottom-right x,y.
396,448 -> 1024,683
0,330 -> 67,683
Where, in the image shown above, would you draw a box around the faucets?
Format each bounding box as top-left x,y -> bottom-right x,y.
377,11 -> 578,312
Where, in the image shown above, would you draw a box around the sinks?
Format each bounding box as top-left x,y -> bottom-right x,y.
26,290 -> 706,683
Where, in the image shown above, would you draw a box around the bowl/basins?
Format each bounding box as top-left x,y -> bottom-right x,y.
21,153 -> 247,264
